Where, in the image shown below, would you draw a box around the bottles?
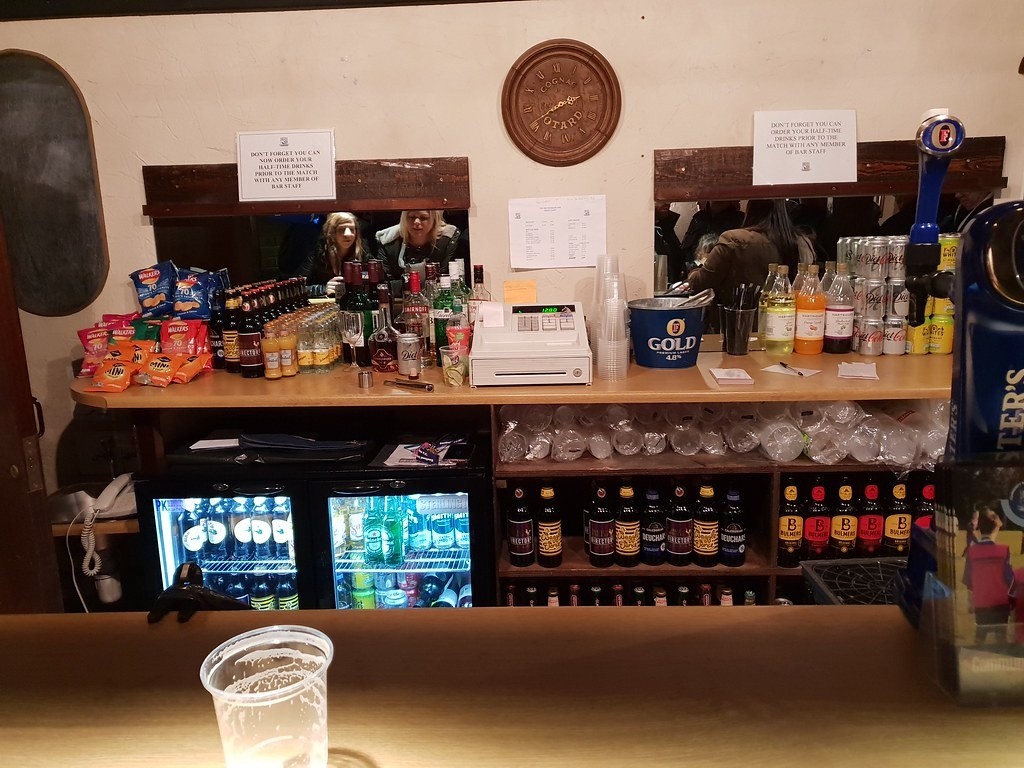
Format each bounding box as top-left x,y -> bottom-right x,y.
756,263 -> 778,347
206,254 -> 492,380
792,263 -> 808,296
824,262 -> 855,353
819,260 -> 837,290
765,265 -> 796,355
504,472 -> 936,611
793,264 -> 826,354
171,498 -> 472,615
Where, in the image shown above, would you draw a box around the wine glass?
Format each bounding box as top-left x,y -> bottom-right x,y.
337,308 -> 365,373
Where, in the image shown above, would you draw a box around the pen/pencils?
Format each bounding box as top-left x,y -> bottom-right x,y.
780,362 -> 804,376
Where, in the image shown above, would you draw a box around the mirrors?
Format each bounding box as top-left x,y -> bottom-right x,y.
654,192 -> 996,352
152,207 -> 472,305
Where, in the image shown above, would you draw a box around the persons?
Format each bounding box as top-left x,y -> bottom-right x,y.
17,138 -> 90,318
375,208 -> 460,281
962,510 -> 979,558
963,506 -> 1015,649
681,200 -> 746,262
299,212 -> 375,296
882,195 -> 918,235
828,199 -> 883,246
950,191 -> 993,234
654,202 -> 688,289
785,201 -> 798,216
792,204 -> 831,262
684,199 -> 816,336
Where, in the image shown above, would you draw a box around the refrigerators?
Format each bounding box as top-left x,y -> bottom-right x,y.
132,425 -> 494,613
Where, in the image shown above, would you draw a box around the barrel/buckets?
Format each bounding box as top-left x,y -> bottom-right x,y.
624,295 -> 710,368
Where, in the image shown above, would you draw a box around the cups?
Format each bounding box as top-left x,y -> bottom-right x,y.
496,399 -> 956,468
438,344 -> 468,388
585,254 -> 632,381
199,625 -> 335,768
723,308 -> 757,356
716,303 -> 726,342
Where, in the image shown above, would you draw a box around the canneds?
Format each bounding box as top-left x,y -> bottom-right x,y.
407,492 -> 470,550
349,552 -> 418,610
775,598 -> 792,605
397,333 -> 422,376
836,232 -> 959,357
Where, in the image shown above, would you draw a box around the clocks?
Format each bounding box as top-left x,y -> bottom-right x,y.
501,38 -> 623,168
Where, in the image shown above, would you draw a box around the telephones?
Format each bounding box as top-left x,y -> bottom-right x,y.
93,472 -> 137,519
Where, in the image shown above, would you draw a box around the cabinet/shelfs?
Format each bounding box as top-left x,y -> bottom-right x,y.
46,404 -> 916,607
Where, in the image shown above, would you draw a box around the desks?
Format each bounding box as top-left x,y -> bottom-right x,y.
0,606 -> 1024,768
